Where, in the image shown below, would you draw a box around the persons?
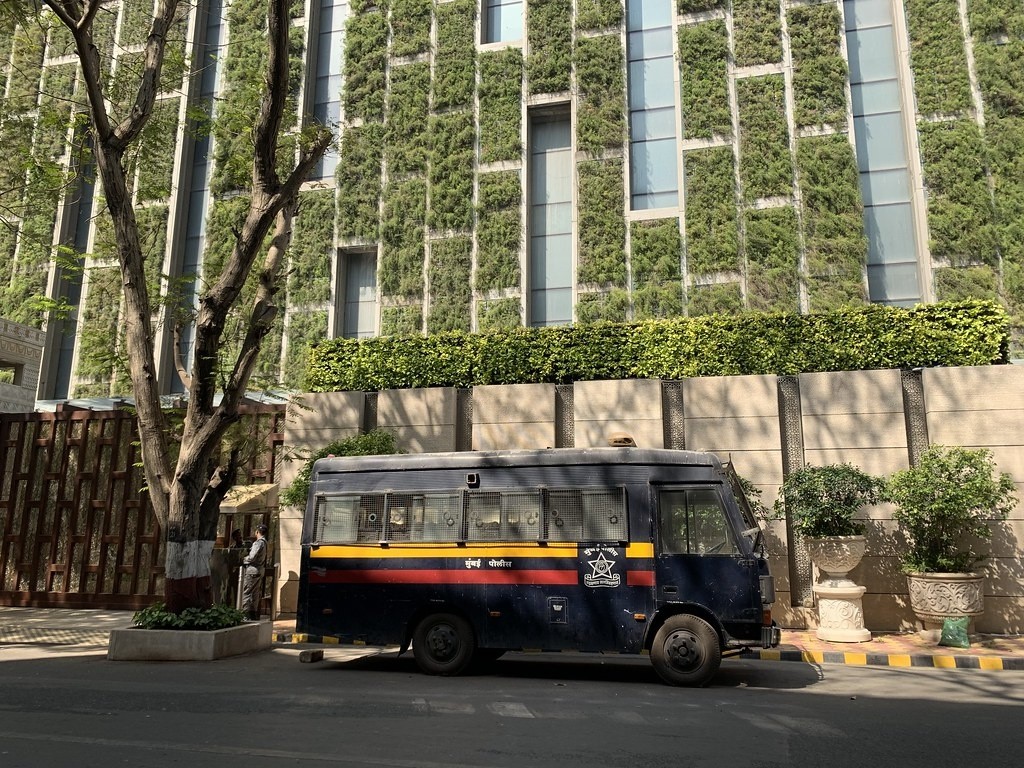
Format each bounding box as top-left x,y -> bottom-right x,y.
239,525 -> 269,622
230,527 -> 247,549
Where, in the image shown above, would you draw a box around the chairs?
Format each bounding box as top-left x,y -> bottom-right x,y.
325,519 -> 597,540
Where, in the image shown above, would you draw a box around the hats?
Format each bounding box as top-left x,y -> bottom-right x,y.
232,529 -> 241,536
255,524 -> 267,531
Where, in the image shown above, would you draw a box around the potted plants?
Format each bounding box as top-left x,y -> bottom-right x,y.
886,444 -> 1020,640
772,462 -> 885,642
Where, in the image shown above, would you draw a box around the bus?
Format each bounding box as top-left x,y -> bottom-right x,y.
289,448 -> 780,684
